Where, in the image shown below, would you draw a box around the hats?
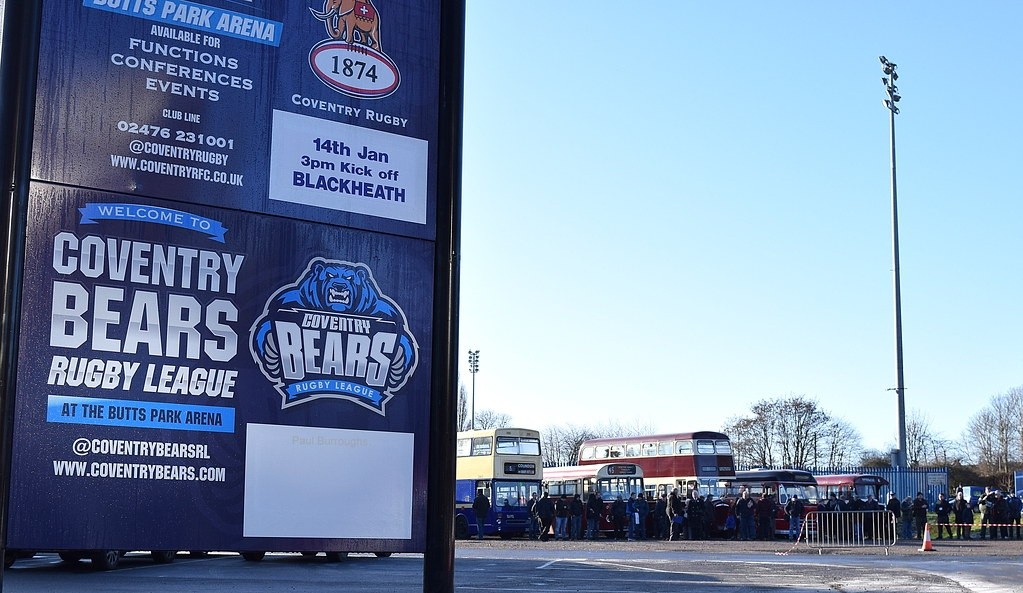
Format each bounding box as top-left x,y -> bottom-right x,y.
793,495 -> 798,499
917,492 -> 923,496
707,494 -> 714,499
560,494 -> 567,499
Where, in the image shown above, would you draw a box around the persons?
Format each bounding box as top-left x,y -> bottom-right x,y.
527,492 -> 604,540
818,491 -> 928,539
978,486 -> 1023,541
655,487 -> 780,542
472,492 -> 490,540
609,492 -> 650,541
935,483 -> 974,540
784,494 -> 805,542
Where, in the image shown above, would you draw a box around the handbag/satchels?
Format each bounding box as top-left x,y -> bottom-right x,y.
672,515 -> 683,523
635,512 -> 639,524
725,516 -> 736,529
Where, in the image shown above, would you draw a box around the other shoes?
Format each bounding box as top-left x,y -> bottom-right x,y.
917,535 -> 1022,541
628,538 -> 635,542
538,536 -> 545,542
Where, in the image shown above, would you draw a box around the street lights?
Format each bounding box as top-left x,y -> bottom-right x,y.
879,54 -> 910,470
467,350 -> 479,429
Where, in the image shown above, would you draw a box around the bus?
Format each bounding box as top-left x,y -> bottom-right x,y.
497,462 -> 645,540
577,431 -> 737,537
808,474 -> 891,524
454,428 -> 542,540
732,468 -> 822,536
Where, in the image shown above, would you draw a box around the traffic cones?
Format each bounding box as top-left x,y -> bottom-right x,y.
918,523 -> 938,552
